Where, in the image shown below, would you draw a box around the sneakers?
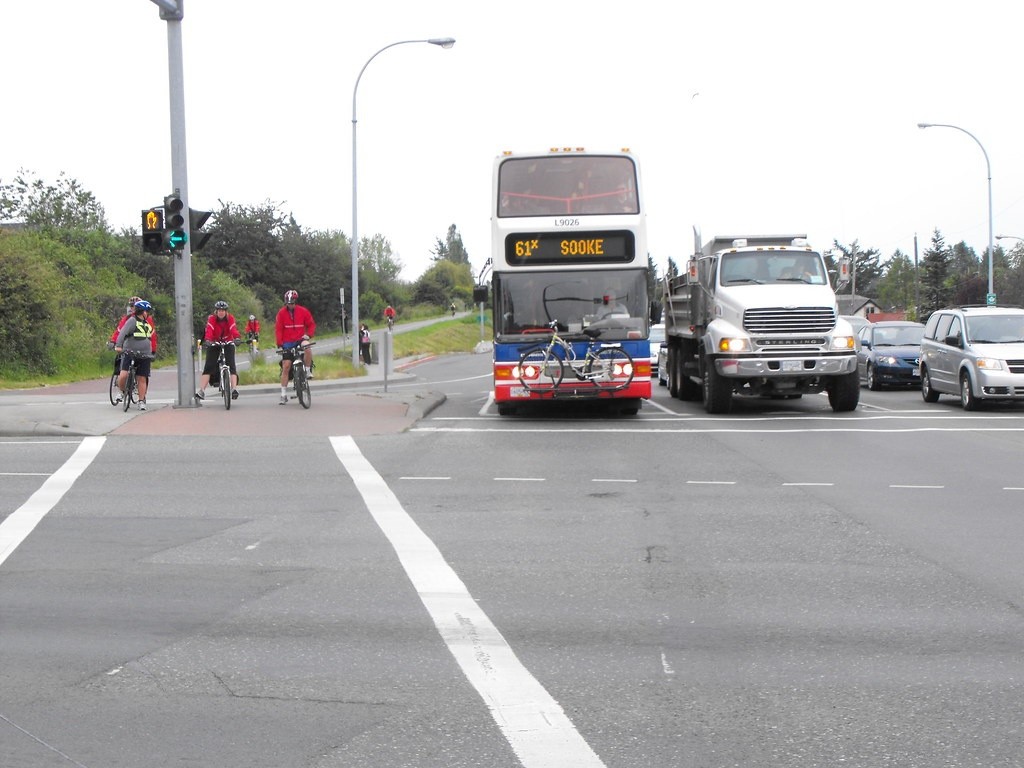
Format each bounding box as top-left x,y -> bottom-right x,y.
232,390 -> 238,400
306,372 -> 314,379
196,390 -> 204,399
138,401 -> 146,410
280,395 -> 288,405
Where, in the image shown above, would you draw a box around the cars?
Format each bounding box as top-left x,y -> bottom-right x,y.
649,325 -> 669,389
839,316 -> 926,391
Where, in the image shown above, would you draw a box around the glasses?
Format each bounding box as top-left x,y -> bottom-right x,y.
287,302 -> 295,305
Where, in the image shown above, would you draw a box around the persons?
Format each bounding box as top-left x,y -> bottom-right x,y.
608,184 -> 635,213
247,315 -> 260,356
196,302 -> 241,400
274,290 -> 317,405
341,309 -> 371,365
597,288 -> 629,321
451,303 -> 456,316
781,259 -> 812,284
385,305 -> 395,331
110,296 -> 156,411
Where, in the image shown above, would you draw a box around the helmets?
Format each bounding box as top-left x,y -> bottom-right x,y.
249,315 -> 256,320
285,289 -> 299,299
215,302 -> 229,309
128,298 -> 141,307
135,300 -> 152,311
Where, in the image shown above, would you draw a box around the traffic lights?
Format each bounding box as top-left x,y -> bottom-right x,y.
141,210 -> 164,252
163,197 -> 188,255
189,208 -> 214,254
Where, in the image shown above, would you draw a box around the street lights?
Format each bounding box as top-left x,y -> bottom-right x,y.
352,38 -> 456,368
917,123 -> 994,294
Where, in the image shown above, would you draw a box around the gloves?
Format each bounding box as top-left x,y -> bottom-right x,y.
234,339 -> 242,347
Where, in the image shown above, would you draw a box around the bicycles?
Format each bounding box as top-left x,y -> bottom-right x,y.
244,333 -> 259,356
106,342 -> 154,412
276,342 -> 316,409
518,319 -> 634,393
203,341 -> 244,410
387,317 -> 394,331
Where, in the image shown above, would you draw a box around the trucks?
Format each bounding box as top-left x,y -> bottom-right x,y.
663,226 -> 860,414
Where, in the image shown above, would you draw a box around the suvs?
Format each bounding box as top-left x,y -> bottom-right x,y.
919,305 -> 1024,411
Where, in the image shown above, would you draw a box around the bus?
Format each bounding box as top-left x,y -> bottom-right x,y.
473,144 -> 663,416
